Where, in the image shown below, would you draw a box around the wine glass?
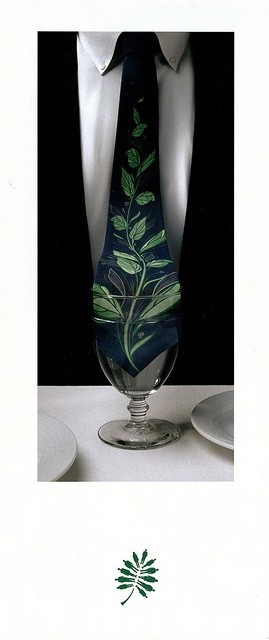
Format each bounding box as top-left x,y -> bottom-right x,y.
93,295 -> 180,449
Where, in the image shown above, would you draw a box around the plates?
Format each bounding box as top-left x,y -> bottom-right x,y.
38,414 -> 78,482
191,392 -> 234,451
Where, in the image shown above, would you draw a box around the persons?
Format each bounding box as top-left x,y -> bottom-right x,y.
38,32 -> 234,385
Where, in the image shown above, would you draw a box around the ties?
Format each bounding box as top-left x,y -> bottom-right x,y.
91,31 -> 182,379
